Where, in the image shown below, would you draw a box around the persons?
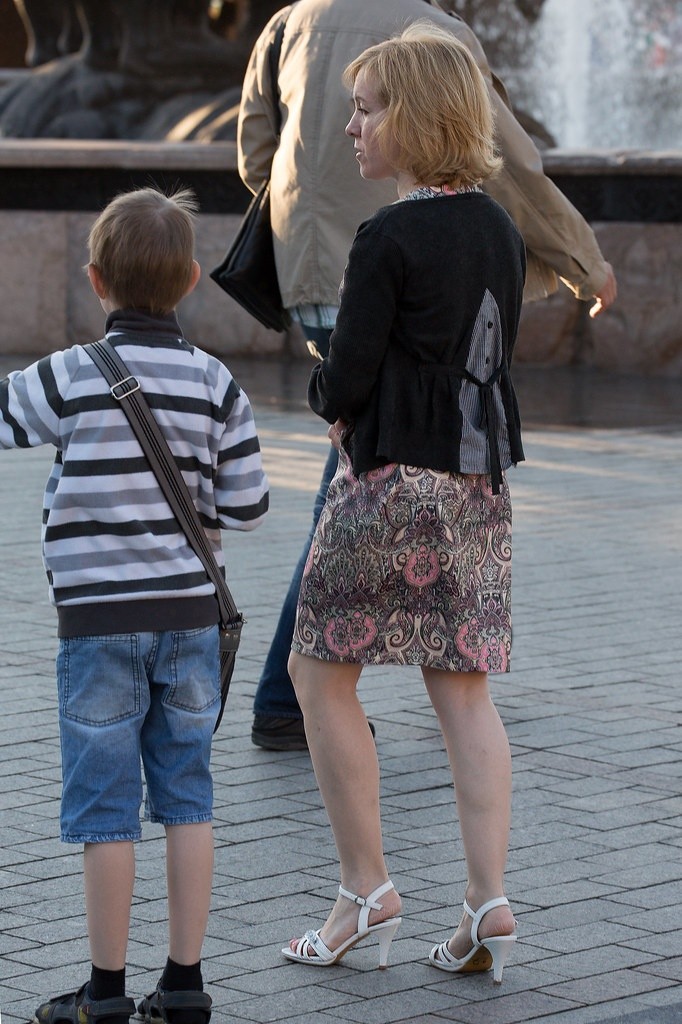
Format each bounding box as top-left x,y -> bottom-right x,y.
282,29 -> 522,984
1,189 -> 271,1024
236,0 -> 617,750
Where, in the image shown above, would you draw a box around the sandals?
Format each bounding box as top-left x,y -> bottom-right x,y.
143,983 -> 212,1024
34,980 -> 137,1024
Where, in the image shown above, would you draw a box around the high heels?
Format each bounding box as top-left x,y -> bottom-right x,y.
281,879 -> 402,970
428,896 -> 517,985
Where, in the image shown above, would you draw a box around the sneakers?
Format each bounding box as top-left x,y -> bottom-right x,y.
252,714 -> 376,750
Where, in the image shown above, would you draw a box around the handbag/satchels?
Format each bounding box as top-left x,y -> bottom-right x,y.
208,176 -> 288,333
211,611 -> 248,734
